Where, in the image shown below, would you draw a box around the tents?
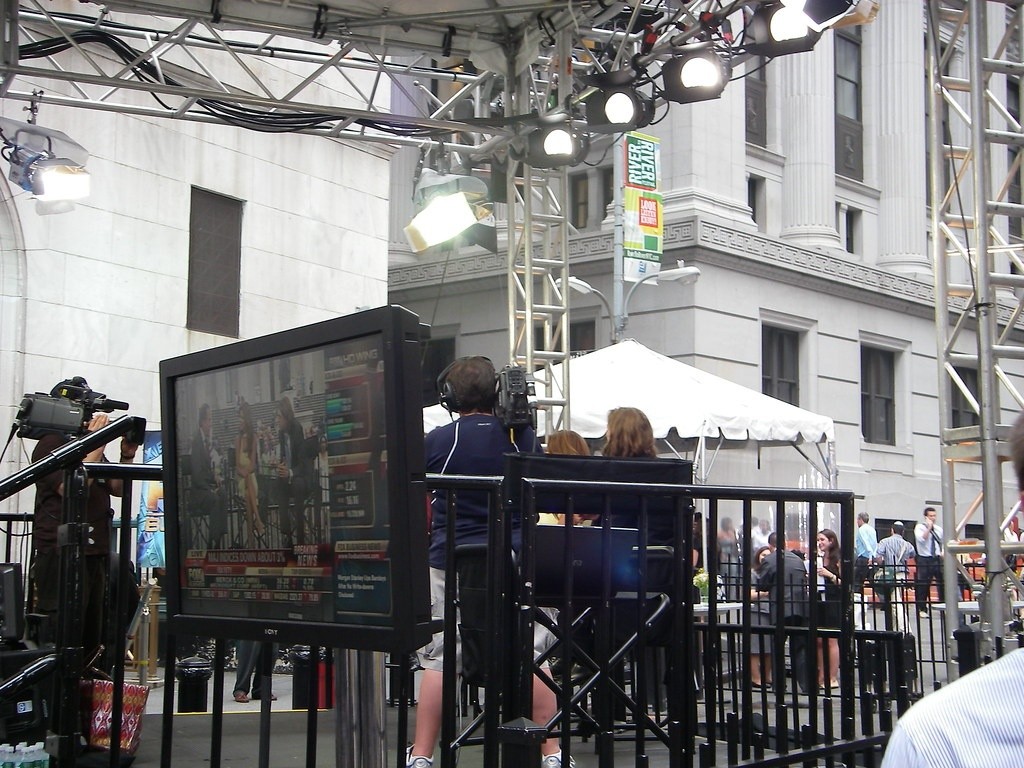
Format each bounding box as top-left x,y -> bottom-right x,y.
424,337 -> 841,544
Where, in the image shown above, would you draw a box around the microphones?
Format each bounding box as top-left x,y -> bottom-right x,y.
93,399 -> 129,410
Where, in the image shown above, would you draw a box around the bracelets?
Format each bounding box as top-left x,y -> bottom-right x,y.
121,452 -> 135,459
831,575 -> 836,582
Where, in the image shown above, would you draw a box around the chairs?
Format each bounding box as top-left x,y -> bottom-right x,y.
454,453 -> 694,768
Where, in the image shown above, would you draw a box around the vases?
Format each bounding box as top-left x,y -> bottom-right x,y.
700,588 -> 708,606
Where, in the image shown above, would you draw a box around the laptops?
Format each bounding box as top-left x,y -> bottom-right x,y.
524,524 -> 641,598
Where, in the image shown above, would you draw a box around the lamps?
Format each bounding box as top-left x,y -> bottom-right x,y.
587,88 -> 655,133
527,125 -> 589,169
743,1 -> 880,58
662,51 -> 731,104
0,116 -> 93,216
403,146 -> 498,257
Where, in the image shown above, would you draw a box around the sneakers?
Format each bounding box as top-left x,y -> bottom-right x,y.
541,749 -> 576,768
406,743 -> 433,768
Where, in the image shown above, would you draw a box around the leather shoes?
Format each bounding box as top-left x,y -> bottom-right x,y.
918,555 -> 942,559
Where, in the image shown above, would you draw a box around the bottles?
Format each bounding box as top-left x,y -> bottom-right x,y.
0,742 -> 50,768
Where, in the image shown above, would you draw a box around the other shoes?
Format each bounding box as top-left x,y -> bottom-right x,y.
252,692 -> 278,700
819,677 -> 841,688
752,681 -> 787,692
920,610 -> 929,618
234,691 -> 249,702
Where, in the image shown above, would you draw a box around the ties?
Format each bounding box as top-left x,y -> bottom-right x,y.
929,524 -> 936,558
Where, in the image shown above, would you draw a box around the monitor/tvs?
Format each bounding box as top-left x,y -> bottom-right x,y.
159,303 -> 433,654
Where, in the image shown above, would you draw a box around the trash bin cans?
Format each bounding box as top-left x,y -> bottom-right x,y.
289,648 -> 336,708
175,661 -> 208,712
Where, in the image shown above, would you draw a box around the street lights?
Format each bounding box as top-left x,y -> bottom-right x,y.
555,265 -> 700,343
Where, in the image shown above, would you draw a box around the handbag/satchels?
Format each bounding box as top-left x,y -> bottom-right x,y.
874,568 -> 894,596
81,679 -> 151,753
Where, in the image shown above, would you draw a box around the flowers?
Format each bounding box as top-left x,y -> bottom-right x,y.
693,569 -> 722,597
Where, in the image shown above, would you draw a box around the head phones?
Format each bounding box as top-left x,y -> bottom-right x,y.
439,355 -> 495,412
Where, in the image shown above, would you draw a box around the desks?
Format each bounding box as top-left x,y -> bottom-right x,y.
692,603 -> 754,690
926,601 -> 1024,661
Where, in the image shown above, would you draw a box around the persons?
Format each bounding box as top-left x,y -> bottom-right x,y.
877,521 -> 915,636
854,512 -> 881,610
881,411 -> 1024,768
234,641 -> 280,702
693,512 -> 843,696
406,356 -> 577,768
538,408 -> 677,712
914,507 -> 944,618
189,397 -> 331,550
1002,516 -> 1024,621
31,381 -> 139,686
